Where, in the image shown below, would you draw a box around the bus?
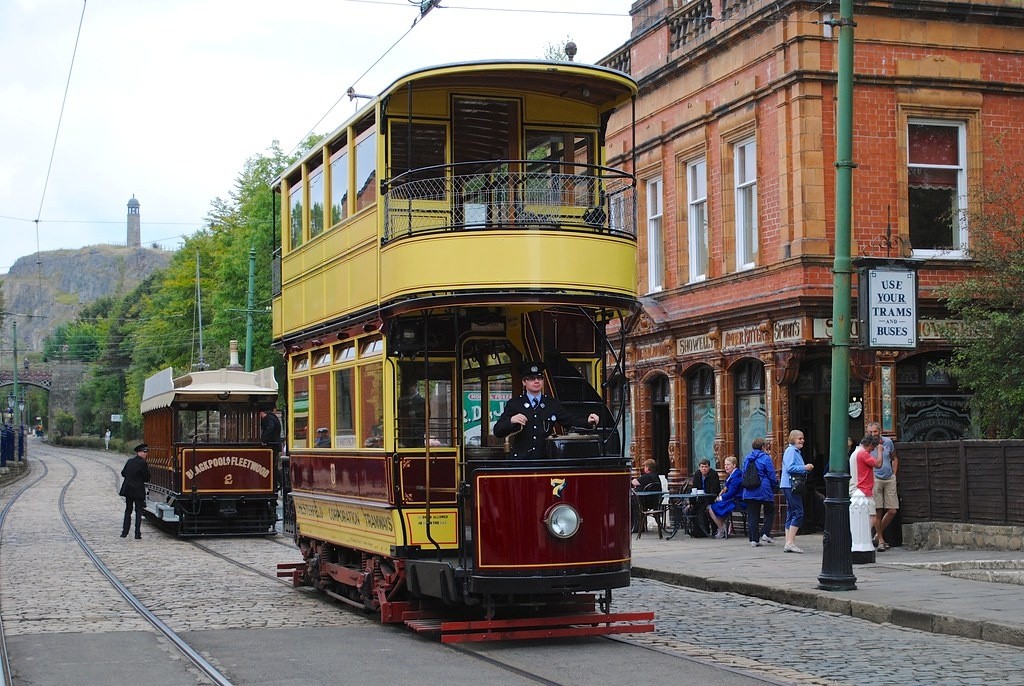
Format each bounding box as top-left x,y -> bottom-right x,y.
138,249 -> 285,539
271,59 -> 654,643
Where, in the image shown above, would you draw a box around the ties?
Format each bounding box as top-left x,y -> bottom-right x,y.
533,397 -> 538,409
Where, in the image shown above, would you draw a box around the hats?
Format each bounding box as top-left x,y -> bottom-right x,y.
133,443 -> 149,453
399,377 -> 417,391
517,362 -> 546,376
316,428 -> 329,434
642,459 -> 657,467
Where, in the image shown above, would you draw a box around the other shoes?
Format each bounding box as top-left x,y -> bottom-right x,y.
877,542 -> 886,551
714,531 -> 724,538
134,535 -> 142,539
784,544 -> 802,553
762,535 -> 774,543
873,538 -> 889,548
121,531 -> 127,538
750,541 -> 761,546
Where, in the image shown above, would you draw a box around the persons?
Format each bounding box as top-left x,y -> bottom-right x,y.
398,378 -> 431,447
780,430 -> 814,553
105,428 -> 111,451
631,458 -> 662,533
683,458 -> 721,535
260,410 -> 280,491
313,428 -> 331,448
823,437 -> 853,475
742,438 -> 778,547
865,421 -> 899,552
119,443 -> 151,539
849,433 -> 883,528
708,456 -> 748,539
492,361 -> 600,467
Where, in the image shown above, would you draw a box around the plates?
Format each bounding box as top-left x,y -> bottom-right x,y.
697,493 -> 706,494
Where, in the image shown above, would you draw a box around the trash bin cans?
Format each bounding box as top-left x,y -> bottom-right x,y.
872,482 -> 903,548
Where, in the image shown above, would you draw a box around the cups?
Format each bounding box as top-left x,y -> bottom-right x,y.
692,488 -> 697,494
697,490 -> 704,493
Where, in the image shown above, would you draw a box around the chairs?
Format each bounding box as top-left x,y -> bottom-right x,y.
630,480 -> 749,540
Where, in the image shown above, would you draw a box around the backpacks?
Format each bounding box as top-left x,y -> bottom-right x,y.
741,454 -> 765,489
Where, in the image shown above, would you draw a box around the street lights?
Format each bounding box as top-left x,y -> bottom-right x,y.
17,393 -> 26,436
6,390 -> 16,430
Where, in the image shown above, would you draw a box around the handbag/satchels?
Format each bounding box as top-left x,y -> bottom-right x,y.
791,474 -> 808,494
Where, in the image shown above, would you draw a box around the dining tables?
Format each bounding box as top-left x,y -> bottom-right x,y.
632,489 -> 669,540
661,494 -> 716,542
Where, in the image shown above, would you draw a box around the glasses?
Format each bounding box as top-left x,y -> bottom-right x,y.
523,376 -> 545,382
